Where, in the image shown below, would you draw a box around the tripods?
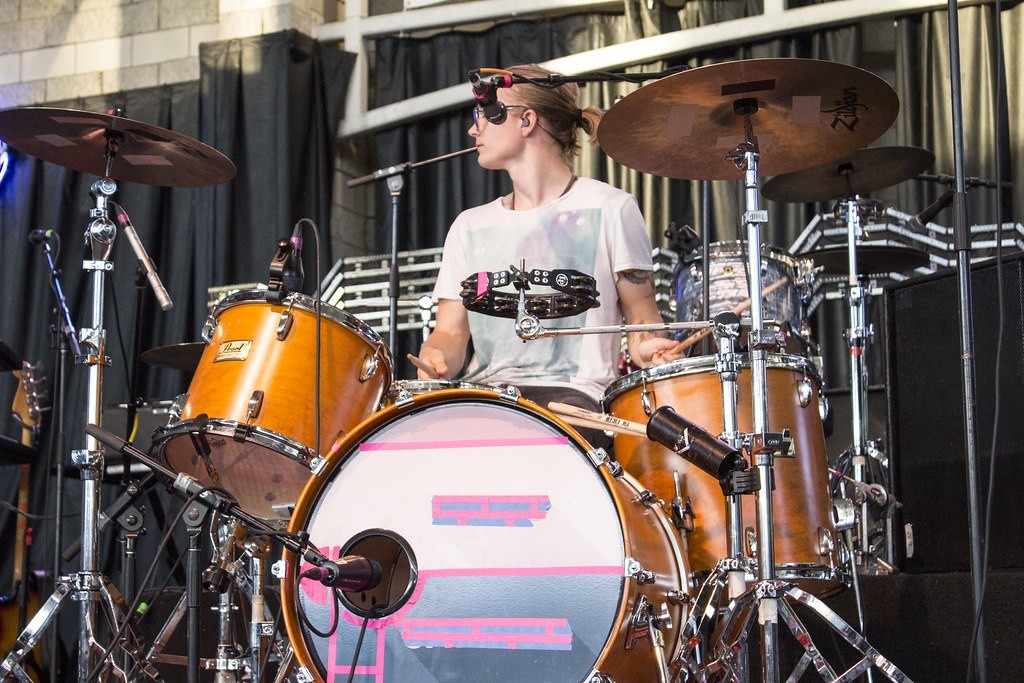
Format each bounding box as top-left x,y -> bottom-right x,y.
0,128 -> 298,683
669,116 -> 910,683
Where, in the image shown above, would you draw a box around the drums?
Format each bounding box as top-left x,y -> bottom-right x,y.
385,377 -> 506,409
595,348 -> 852,606
273,387 -> 696,683
666,237 -> 809,356
157,287 -> 394,528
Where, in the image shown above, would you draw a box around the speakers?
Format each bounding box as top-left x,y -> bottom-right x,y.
878,252 -> 1024,571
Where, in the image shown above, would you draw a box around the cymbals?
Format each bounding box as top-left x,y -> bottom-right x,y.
790,243 -> 930,276
0,105 -> 238,189
596,57 -> 901,181
140,340 -> 207,374
760,145 -> 937,204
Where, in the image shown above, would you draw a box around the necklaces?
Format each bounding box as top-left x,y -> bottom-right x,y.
511,176 -> 577,212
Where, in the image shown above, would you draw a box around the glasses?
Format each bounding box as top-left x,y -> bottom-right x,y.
473,102 -> 539,125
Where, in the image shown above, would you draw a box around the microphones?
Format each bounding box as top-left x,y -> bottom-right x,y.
283,223 -> 304,292
305,554 -> 383,592
468,72 -> 507,125
28,229 -> 53,245
114,205 -> 172,310
908,188 -> 956,233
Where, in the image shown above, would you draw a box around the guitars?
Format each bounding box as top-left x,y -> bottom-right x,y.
1,359 -> 53,682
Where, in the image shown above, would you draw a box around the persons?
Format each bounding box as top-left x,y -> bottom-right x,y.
417,64 -> 686,450
